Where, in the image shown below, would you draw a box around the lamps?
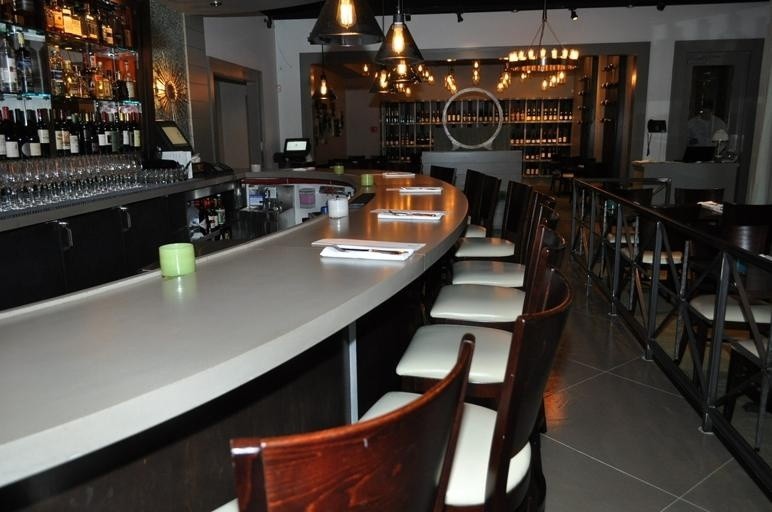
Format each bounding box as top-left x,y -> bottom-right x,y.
374,0 -> 425,67
569,8 -> 578,20
455,13 -> 464,24
406,15 -> 412,22
506,20 -> 581,73
264,17 -> 274,30
306,0 -> 386,47
312,74 -> 336,102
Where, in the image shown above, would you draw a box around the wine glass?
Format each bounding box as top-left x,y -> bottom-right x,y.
0,150 -> 188,212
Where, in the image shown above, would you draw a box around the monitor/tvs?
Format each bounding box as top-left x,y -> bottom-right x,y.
157,117 -> 193,156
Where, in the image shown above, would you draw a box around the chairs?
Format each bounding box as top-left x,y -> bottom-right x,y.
599,187 -> 681,304
722,199 -> 772,215
673,185 -> 726,202
620,203 -> 708,342
723,336 -> 772,425
690,214 -> 772,390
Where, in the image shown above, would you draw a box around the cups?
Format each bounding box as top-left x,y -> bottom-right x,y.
333,166 -> 345,175
158,243 -> 197,280
251,164 -> 261,173
361,174 -> 374,186
328,198 -> 349,220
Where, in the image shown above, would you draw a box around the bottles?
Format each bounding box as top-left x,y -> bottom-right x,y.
266,190 -> 288,214
347,192 -> 351,200
0,0 -> 143,160
385,107 -> 572,176
187,193 -> 231,242
234,180 -> 243,209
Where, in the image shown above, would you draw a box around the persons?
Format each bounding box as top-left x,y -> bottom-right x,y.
683,105 -> 726,162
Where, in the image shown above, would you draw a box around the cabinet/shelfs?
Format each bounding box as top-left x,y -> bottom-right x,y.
0,0 -> 245,312
379,95 -> 574,180
